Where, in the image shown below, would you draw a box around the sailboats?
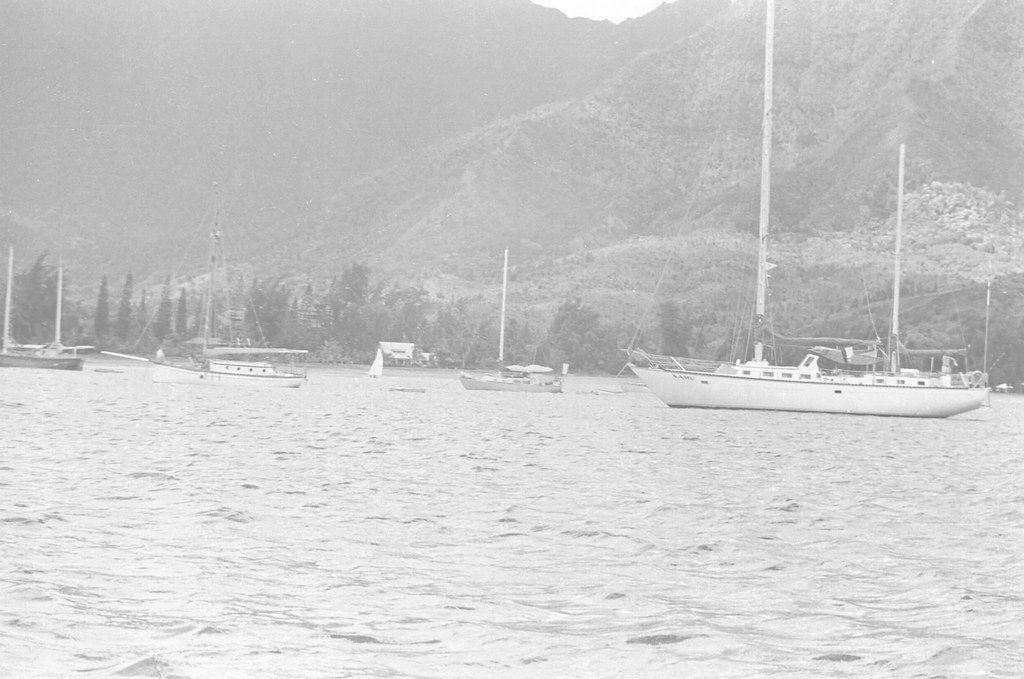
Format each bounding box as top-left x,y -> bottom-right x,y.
457,250 -> 563,393
625,0 -> 995,418
369,348 -> 383,378
100,182 -> 310,389
0,244 -> 95,370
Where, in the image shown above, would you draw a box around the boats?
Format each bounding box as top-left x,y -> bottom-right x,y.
395,386 -> 426,393
